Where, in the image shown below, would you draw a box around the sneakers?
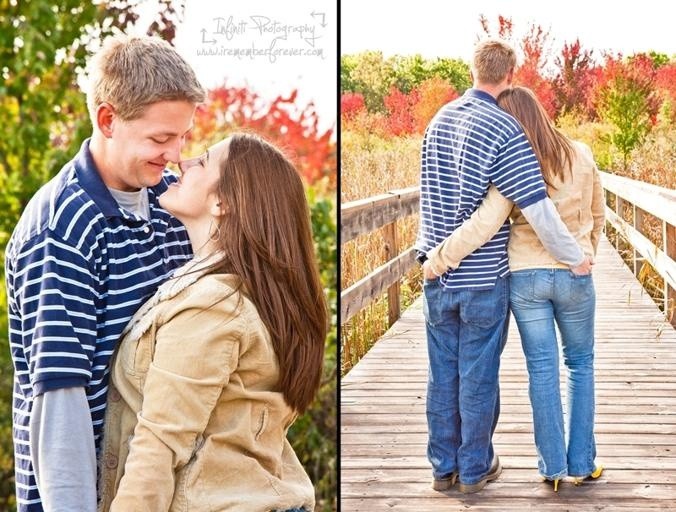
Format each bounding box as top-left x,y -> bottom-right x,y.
432,471 -> 460,491
459,452 -> 503,494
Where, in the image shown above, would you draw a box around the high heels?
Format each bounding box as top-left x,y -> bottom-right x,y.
540,472 -> 562,492
572,464 -> 604,485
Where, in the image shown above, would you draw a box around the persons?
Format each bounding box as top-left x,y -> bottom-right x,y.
420,89 -> 605,493
94,135 -> 331,512
412,40 -> 593,495
6,37 -> 199,512
